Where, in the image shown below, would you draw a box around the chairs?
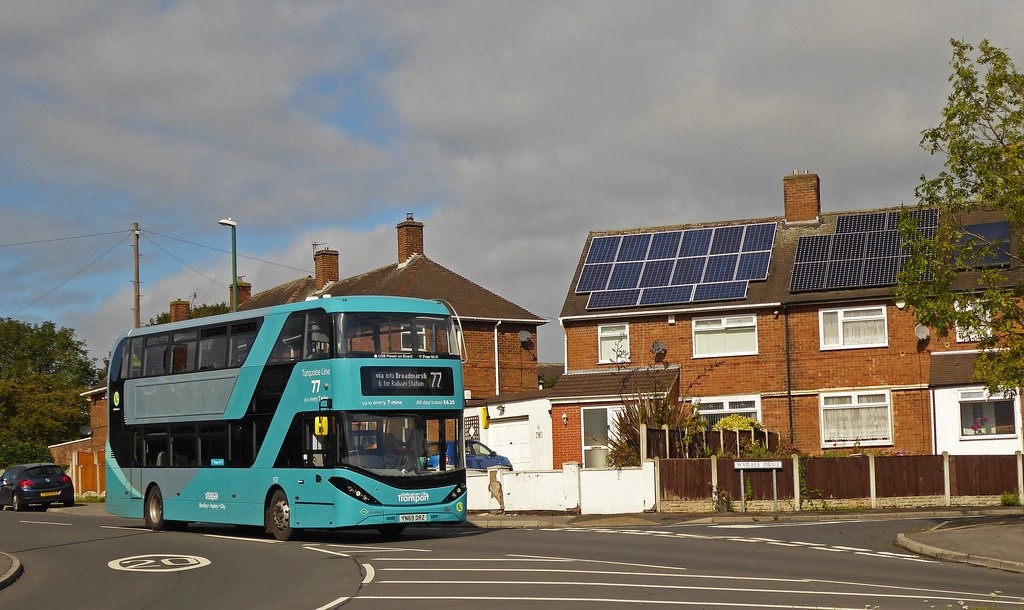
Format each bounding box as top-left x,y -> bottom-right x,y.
152,368 -> 164,375
182,364 -> 193,372
157,451 -> 166,466
213,360 -> 226,369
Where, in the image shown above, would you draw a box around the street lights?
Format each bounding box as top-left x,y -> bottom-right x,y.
217,218 -> 238,311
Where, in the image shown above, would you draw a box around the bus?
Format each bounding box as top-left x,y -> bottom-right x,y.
103,294 -> 490,540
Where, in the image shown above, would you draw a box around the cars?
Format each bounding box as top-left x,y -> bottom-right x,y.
425,440 -> 515,471
0,462 -> 76,511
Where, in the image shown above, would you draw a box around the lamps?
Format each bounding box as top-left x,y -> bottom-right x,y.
497,405 -> 505,411
